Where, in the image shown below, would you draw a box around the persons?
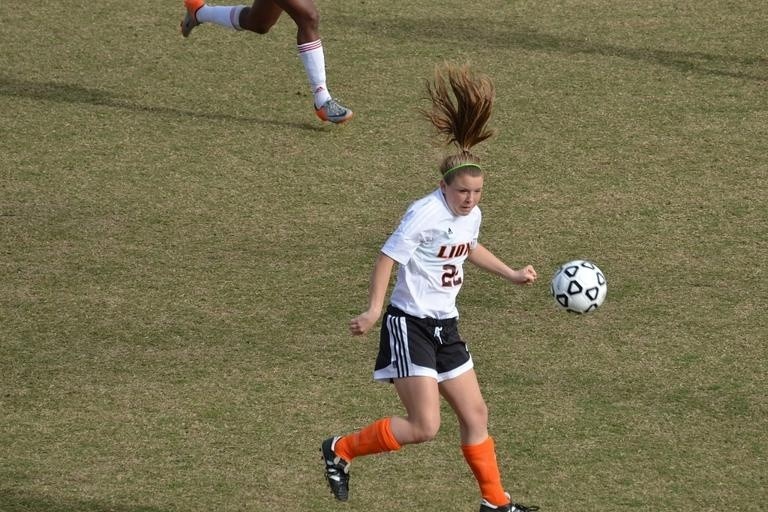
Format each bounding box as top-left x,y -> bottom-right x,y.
319,68 -> 541,510
180,0 -> 355,123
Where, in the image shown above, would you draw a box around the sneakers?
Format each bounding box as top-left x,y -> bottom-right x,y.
313,98 -> 355,124
179,0 -> 205,38
479,492 -> 539,512
320,436 -> 350,502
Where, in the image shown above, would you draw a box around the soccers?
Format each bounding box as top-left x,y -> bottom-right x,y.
550,261 -> 607,315
